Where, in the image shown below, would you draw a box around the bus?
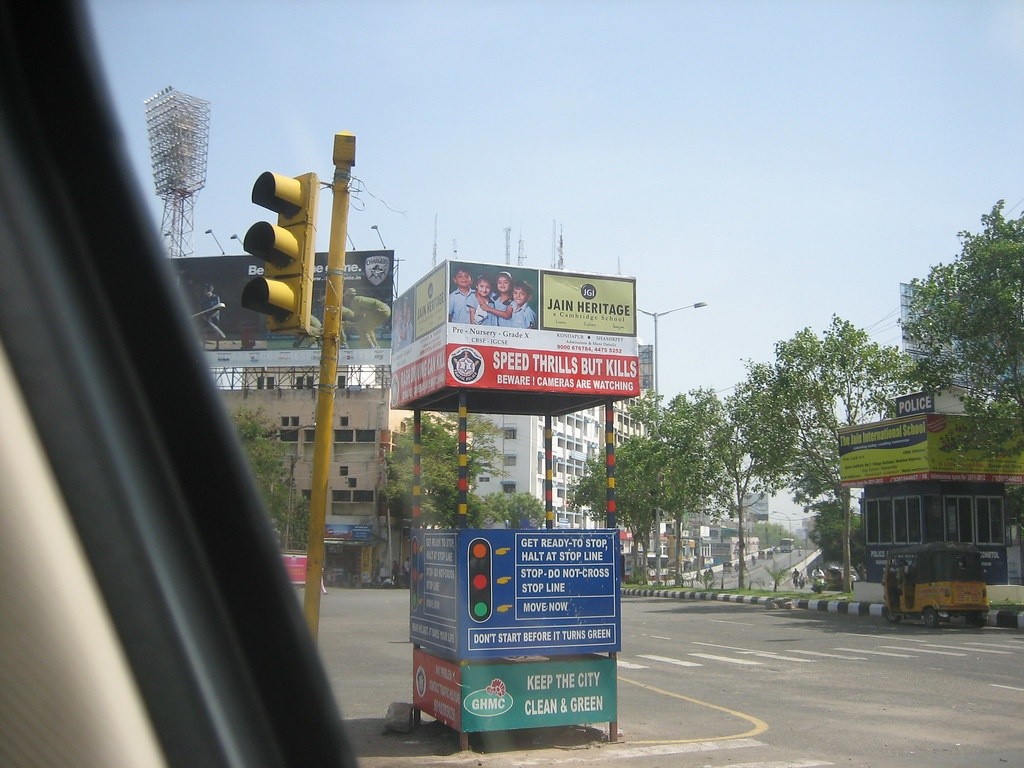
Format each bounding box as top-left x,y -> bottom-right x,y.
780,537 -> 795,552
622,552 -> 669,583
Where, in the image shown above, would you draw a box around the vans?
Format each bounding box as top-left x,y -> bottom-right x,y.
756,548 -> 773,561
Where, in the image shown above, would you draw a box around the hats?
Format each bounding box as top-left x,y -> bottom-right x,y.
205,283 -> 214,293
345,288 -> 357,296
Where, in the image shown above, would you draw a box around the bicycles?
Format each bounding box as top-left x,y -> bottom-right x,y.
793,577 -> 799,589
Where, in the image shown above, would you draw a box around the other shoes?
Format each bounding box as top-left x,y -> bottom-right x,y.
340,342 -> 347,349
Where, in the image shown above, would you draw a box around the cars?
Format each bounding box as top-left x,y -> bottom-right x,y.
826,566 -> 859,591
665,566 -> 676,580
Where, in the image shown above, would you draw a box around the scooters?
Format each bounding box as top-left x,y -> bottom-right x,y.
709,572 -> 712,580
810,575 -> 825,594
799,580 -> 806,589
734,566 -> 739,572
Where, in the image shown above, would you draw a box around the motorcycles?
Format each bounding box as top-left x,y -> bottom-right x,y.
881,541 -> 989,628
722,561 -> 732,573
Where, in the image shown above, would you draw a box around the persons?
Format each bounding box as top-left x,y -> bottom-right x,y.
392,300 -> 413,352
449,268 -> 537,329
199,283 -> 220,350
403,557 -> 410,572
704,570 -> 708,574
709,567 -> 713,572
293,313 -> 322,348
320,567 -> 328,594
812,566 -> 824,592
393,561 -> 400,584
341,288 -> 391,349
799,573 -> 805,580
792,568 -> 799,587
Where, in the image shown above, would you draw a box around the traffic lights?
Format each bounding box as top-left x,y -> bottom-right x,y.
240,170 -> 321,334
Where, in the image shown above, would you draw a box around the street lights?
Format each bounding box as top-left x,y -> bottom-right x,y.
772,511 -> 792,577
637,301 -> 707,586
792,512 -> 806,566
